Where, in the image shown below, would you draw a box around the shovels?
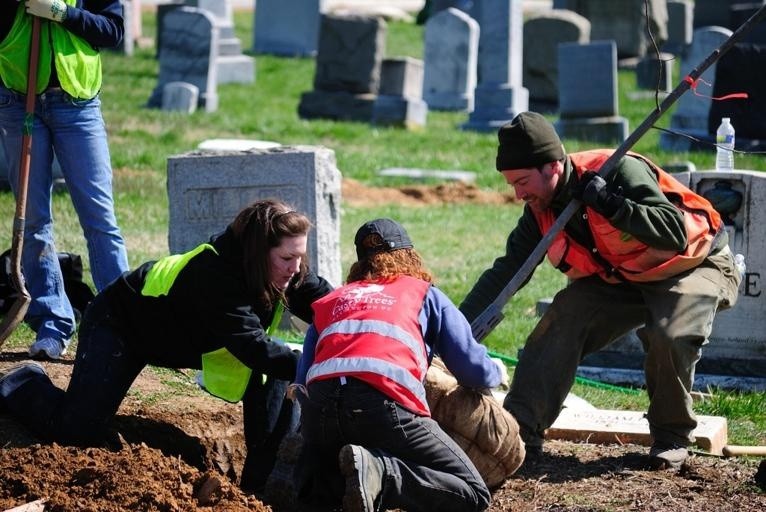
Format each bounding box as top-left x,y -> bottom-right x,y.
0,14 -> 41,346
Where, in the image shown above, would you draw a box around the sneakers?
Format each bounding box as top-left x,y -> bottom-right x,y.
338,444 -> 384,512
0,363 -> 45,397
29,337 -> 68,359
649,440 -> 690,469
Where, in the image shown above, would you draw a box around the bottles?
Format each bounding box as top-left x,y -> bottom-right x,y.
735,253 -> 747,278
716,117 -> 735,171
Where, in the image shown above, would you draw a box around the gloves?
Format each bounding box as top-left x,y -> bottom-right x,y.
572,170 -> 626,219
25,0 -> 68,23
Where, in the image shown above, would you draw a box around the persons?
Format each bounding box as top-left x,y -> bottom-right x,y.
455,109 -> 743,471
257,215 -> 510,512
0,1 -> 132,365
0,194 -> 346,445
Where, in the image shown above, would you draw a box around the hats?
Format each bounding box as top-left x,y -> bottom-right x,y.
496,111 -> 566,171
354,218 -> 414,261
